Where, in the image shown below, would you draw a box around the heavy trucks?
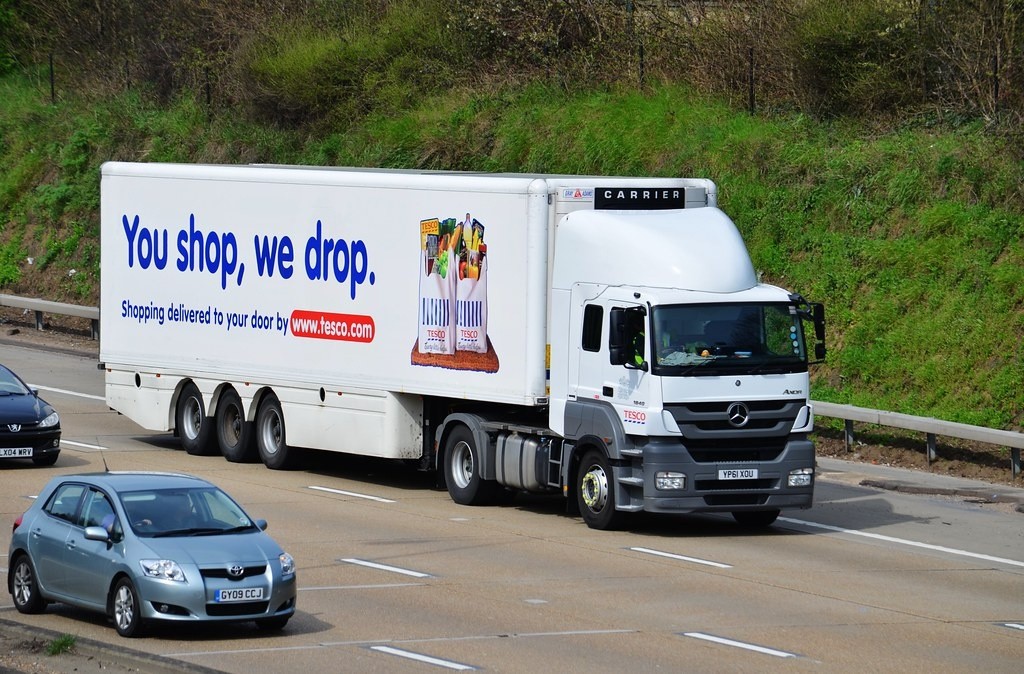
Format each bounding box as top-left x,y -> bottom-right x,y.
101,160 -> 828,531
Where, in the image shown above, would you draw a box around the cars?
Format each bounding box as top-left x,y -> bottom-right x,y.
0,364 -> 62,465
8,470 -> 297,639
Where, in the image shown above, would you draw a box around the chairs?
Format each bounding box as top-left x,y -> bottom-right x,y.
61,496 -> 80,520
704,320 -> 759,346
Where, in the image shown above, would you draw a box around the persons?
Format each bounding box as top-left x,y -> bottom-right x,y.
633,327 -> 668,370
